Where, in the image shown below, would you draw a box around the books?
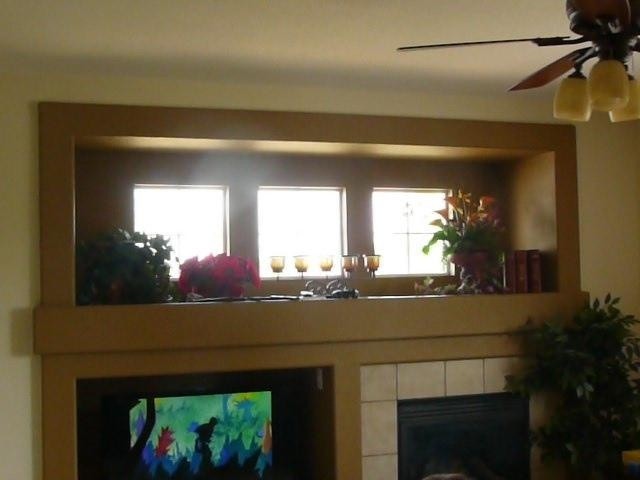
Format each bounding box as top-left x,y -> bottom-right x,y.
503,249 -> 543,294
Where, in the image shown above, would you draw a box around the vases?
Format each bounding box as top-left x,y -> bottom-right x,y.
449,252 -> 500,294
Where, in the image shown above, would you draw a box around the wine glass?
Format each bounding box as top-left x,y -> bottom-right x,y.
270,255 -> 380,278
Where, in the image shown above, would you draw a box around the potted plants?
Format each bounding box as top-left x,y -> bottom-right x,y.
77,223 -> 176,305
503,293 -> 640,480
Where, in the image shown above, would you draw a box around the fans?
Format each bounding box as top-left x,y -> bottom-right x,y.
397,0 -> 640,93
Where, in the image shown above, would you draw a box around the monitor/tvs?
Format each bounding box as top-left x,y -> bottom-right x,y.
102,378 -> 312,480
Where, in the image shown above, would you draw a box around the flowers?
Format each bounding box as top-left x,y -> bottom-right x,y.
423,188 -> 506,257
178,251 -> 261,299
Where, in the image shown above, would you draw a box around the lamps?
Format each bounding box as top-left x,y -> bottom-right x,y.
552,34 -> 640,122
